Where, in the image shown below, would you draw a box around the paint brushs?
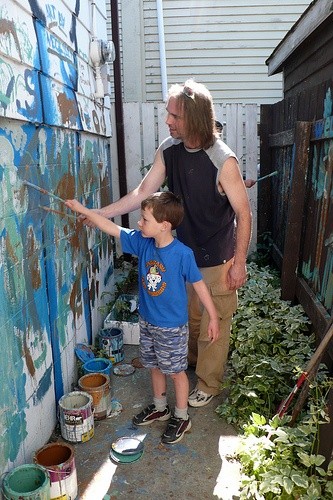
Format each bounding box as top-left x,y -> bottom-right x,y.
24,181 -> 67,204
38,204 -> 77,220
254,170 -> 279,183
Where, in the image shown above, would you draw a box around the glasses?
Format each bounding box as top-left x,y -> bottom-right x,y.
181,83 -> 196,104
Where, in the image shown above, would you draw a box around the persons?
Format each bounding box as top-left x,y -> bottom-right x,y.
214,121 -> 255,188
65,190 -> 220,444
77,81 -> 251,408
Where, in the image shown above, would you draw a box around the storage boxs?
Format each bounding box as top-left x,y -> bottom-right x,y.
103,294 -> 141,345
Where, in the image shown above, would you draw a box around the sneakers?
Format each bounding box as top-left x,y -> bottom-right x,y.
161,412 -> 192,443
188,389 -> 215,407
133,403 -> 170,426
